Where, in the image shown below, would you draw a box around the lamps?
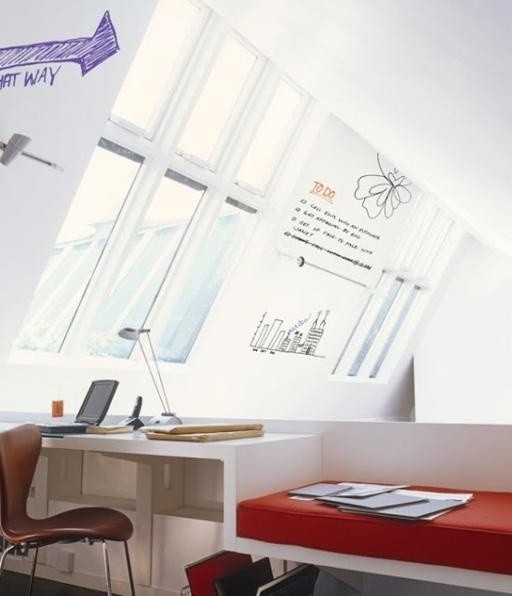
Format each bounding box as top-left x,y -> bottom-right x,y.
114,326 -> 185,428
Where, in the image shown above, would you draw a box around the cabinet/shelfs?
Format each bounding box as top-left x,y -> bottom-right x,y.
1,429 -> 510,596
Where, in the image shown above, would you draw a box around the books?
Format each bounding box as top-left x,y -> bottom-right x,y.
87,425 -> 134,435
215,558 -> 274,596
137,423 -> 266,443
185,552 -> 253,596
289,483 -> 475,523
255,562 -> 320,596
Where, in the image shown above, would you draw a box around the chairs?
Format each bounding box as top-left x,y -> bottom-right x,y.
0,420 -> 145,596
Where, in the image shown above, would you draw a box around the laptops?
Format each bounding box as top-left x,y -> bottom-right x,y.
35,379 -> 119,433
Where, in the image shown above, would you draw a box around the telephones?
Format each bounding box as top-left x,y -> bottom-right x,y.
119,396 -> 144,431
